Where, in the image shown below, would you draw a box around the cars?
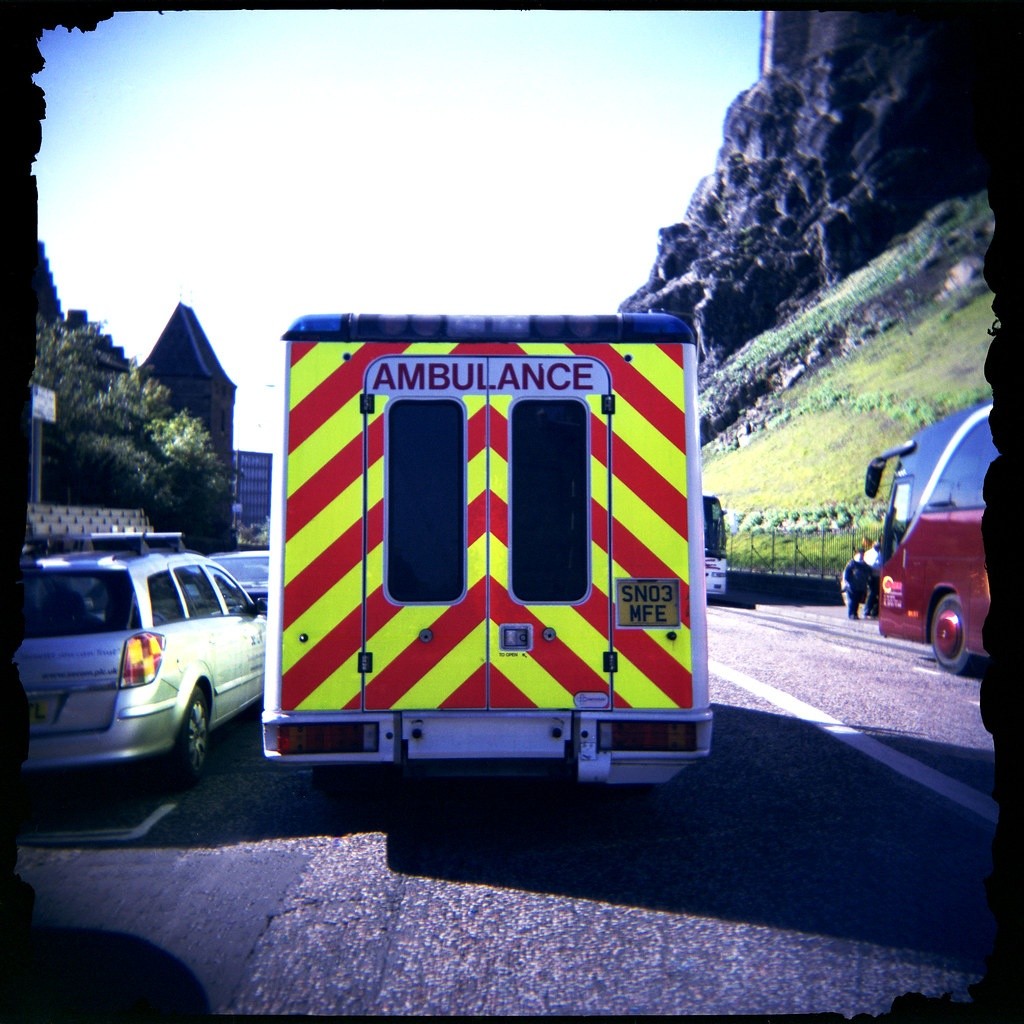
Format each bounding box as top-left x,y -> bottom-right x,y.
839,529 -> 884,623
199,550 -> 270,613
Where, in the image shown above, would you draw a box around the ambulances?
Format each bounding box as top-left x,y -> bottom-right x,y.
260,311 -> 715,799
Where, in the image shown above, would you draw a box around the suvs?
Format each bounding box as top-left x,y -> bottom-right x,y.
13,528 -> 269,788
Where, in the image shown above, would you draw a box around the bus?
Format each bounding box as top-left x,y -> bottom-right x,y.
703,491 -> 727,604
866,395 -> 1003,676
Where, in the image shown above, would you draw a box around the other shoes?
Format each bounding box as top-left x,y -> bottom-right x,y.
864,615 -> 875,620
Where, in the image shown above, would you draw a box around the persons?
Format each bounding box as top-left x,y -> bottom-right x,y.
844,548 -> 871,620
863,534 -> 881,618
